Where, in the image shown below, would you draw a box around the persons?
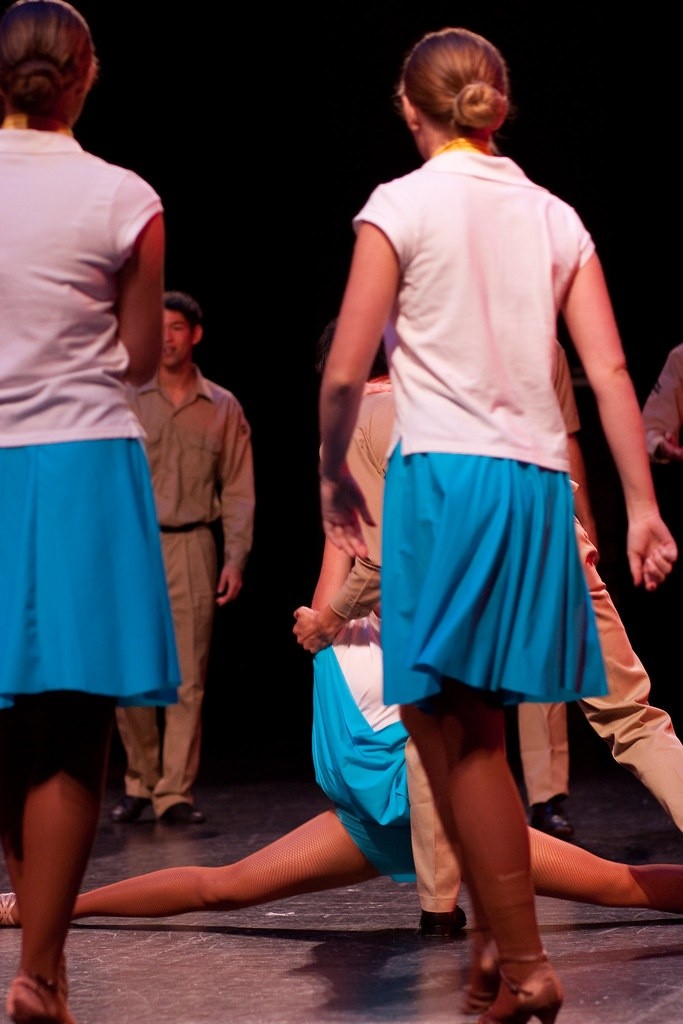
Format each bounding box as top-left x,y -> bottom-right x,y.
1,0 -> 184,1024
295,379 -> 683,860
111,291 -> 254,834
1,638 -> 683,926
640,336 -> 683,463
321,31 -> 678,1024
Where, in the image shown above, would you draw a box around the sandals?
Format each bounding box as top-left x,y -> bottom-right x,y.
0,892 -> 21,926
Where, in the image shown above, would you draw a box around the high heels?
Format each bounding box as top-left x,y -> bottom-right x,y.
7,978 -> 76,1024
479,968 -> 564,1024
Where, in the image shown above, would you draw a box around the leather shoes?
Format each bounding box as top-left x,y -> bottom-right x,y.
531,802 -> 576,840
162,803 -> 205,825
421,905 -> 467,940
109,795 -> 152,822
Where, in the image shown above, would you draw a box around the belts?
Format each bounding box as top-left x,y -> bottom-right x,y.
159,522 -> 203,533
